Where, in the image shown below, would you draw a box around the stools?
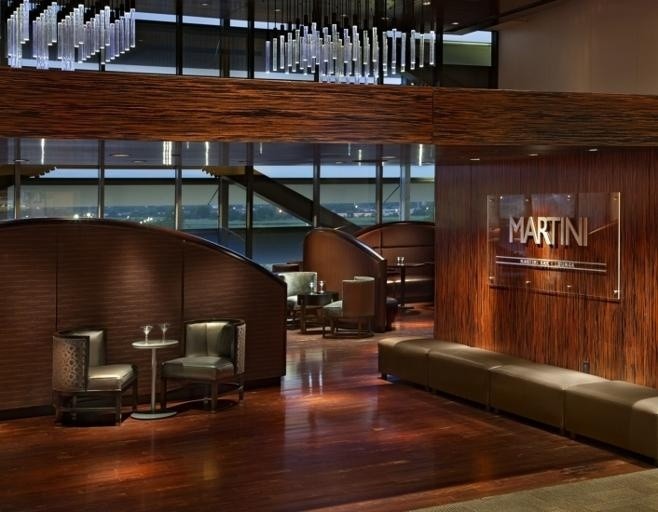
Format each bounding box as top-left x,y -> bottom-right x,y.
379,336 -> 658,463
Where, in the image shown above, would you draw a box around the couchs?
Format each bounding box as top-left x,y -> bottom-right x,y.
387,263 -> 435,328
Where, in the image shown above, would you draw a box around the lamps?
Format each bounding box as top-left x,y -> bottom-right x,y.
1,3 -> 142,77
258,0 -> 436,84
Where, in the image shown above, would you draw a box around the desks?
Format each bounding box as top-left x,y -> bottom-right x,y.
387,263 -> 427,310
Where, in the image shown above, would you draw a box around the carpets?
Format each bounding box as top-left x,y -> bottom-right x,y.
400,466 -> 658,512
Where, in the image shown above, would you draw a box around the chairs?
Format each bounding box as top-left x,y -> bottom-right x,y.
51,318 -> 247,427
271,272 -> 376,339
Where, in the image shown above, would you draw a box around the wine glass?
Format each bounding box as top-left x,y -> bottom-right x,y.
140,326 -> 154,344
308,281 -> 325,295
396,257 -> 405,265
158,323 -> 171,343
